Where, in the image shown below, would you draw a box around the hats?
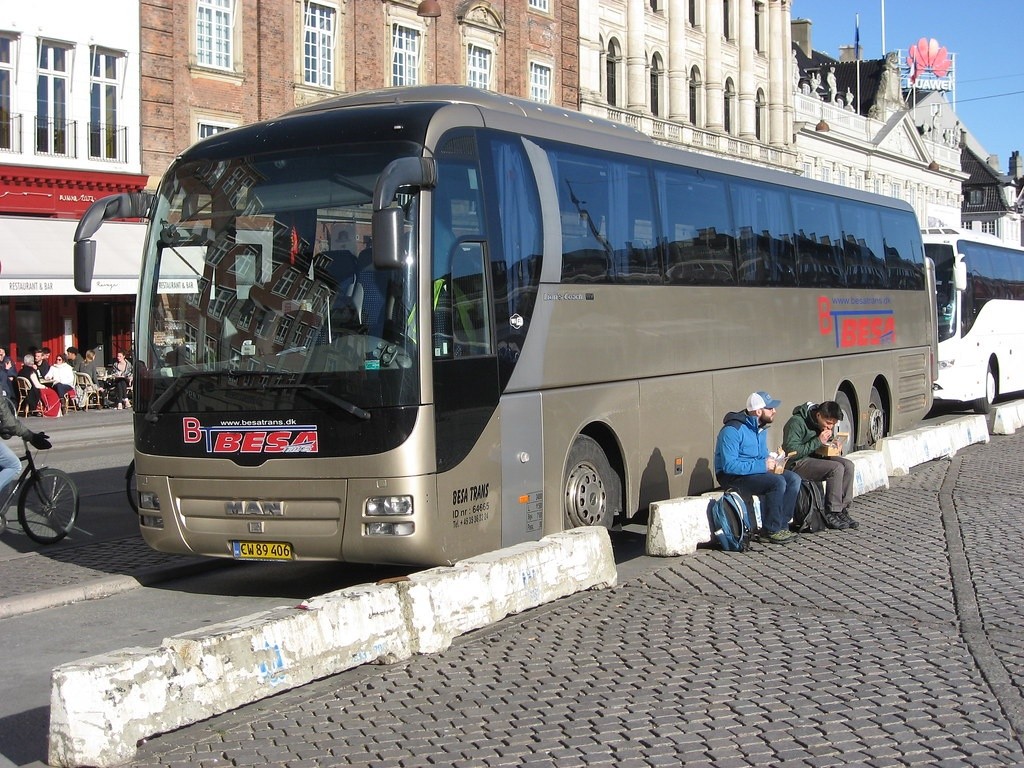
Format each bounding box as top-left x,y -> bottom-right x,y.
747,391 -> 780,411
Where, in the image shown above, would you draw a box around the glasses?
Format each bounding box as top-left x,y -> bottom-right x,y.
57,359 -> 61,361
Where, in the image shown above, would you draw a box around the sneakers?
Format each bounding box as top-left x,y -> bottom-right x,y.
825,509 -> 860,530
758,528 -> 800,544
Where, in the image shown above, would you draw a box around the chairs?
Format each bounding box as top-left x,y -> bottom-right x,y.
15,376 -> 46,420
318,220 -> 929,294
59,373 -> 78,415
74,372 -> 101,412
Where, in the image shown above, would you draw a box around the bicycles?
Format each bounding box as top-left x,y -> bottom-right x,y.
126,458 -> 141,515
0,433 -> 81,546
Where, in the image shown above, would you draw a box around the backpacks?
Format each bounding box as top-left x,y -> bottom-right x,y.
790,480 -> 829,533
712,488 -> 752,553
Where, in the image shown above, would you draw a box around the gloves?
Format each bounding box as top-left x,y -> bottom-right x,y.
29,434 -> 53,450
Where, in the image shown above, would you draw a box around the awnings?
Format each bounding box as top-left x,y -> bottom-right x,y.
0,215 -> 215,297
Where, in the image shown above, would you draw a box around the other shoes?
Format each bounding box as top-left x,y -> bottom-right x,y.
117,401 -> 131,410
102,398 -> 116,406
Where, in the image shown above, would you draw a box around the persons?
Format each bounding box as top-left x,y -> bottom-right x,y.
63,347 -> 87,375
0,347 -> 18,382
715,391 -> 803,544
793,49 -> 801,91
111,351 -> 132,410
781,400 -> 860,531
0,386 -> 52,493
826,67 -> 838,106
33,350 -> 50,381
933,111 -> 943,142
17,354 -> 63,418
953,120 -> 961,150
45,354 -> 75,415
80,350 -> 113,409
41,347 -> 51,360
373,233 -> 476,370
0,357 -> 18,414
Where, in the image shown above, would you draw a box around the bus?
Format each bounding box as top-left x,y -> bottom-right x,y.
915,225 -> 1024,415
72,84 -> 936,573
